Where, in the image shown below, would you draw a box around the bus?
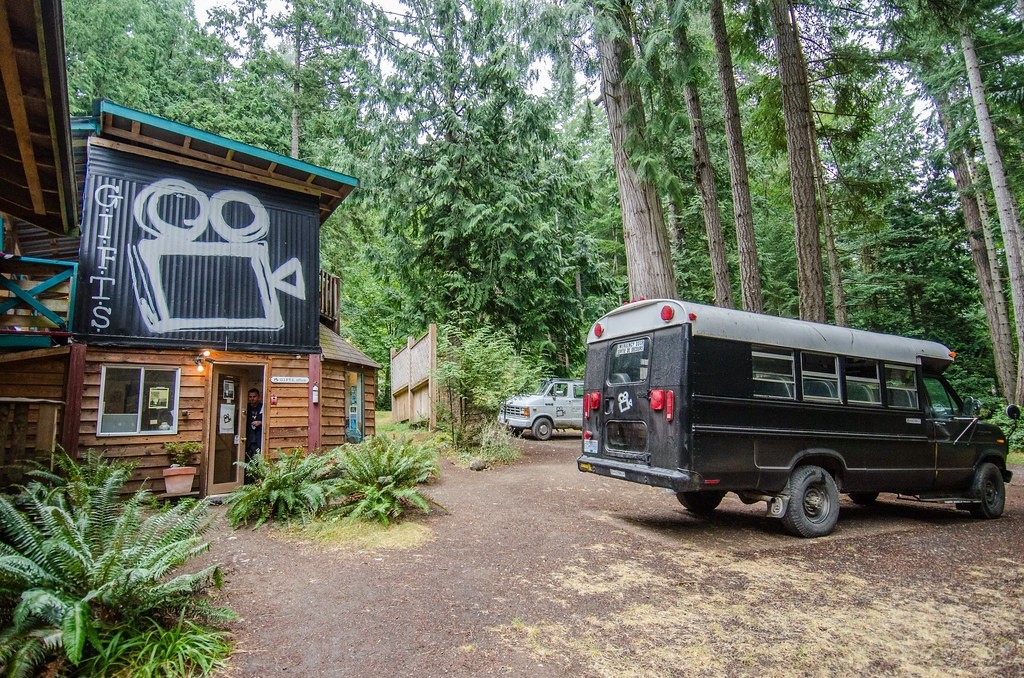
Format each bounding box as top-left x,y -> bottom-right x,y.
577,296 -> 1020,538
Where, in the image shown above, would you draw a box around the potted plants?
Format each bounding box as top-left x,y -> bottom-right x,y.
160,439 -> 204,493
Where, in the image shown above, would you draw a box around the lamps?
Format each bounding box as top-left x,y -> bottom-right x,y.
194,351 -> 215,371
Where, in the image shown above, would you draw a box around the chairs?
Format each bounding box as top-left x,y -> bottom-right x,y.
609,372 -> 631,383
564,386 -> 576,398
752,375 -> 916,408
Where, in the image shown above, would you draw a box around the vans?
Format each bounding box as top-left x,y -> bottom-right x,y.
497,378 -> 584,440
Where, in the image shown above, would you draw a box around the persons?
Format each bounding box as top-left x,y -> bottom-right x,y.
244,388 -> 263,485
